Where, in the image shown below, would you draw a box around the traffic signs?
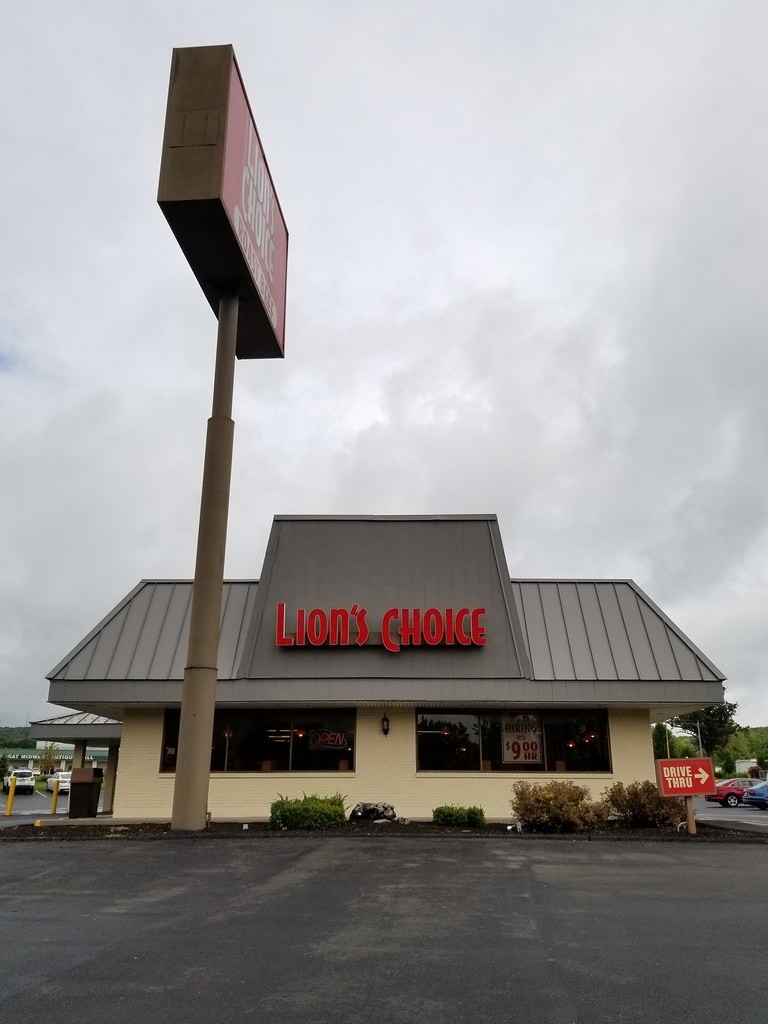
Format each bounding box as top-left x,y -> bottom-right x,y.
654,756 -> 717,797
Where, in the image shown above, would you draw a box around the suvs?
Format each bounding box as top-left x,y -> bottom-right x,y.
2,767 -> 36,796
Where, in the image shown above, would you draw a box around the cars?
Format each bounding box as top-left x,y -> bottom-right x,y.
45,771 -> 72,794
704,777 -> 768,811
32,767 -> 41,776
